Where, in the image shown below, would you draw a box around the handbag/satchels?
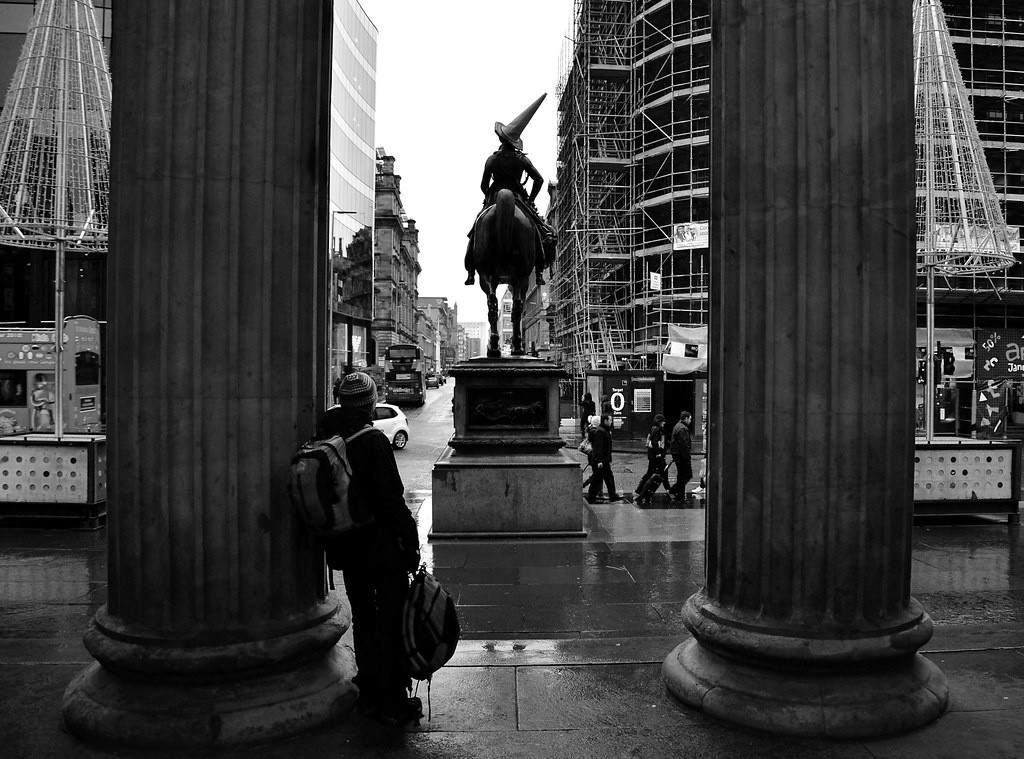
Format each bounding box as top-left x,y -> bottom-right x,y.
646,427 -> 665,452
577,437 -> 593,456
401,565 -> 461,679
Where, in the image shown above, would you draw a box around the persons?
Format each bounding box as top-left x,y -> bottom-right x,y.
691,453 -> 706,493
313,371 -> 423,720
463,133 -> 546,286
675,226 -> 686,243
586,413 -> 623,505
667,411 -> 693,503
633,414 -> 671,496
578,392 -> 596,440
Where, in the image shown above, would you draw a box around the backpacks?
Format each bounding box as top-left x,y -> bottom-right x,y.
288,425 -> 381,540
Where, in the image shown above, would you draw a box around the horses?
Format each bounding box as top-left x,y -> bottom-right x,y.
467,185 -> 538,364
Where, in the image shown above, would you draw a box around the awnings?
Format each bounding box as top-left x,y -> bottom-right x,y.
916,328 -> 973,347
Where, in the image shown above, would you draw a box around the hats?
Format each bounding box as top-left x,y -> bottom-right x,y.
338,372 -> 378,408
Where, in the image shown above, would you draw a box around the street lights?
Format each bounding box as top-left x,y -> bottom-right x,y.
328,211 -> 358,407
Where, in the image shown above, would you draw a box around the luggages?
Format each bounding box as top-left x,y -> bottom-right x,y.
635,460 -> 674,508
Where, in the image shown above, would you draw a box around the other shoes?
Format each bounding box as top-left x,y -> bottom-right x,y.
589,498 -> 604,504
635,487 -> 642,494
669,486 -> 686,503
595,495 -> 610,500
610,495 -> 623,502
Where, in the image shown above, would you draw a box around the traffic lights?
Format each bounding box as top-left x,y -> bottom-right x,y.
944,350 -> 955,375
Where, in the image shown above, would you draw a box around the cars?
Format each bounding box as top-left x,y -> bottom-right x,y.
434,374 -> 443,385
442,376 -> 446,383
327,403 -> 410,451
426,375 -> 439,389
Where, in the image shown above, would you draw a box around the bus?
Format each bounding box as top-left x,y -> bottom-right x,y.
1,315 -> 101,438
384,344 -> 426,407
